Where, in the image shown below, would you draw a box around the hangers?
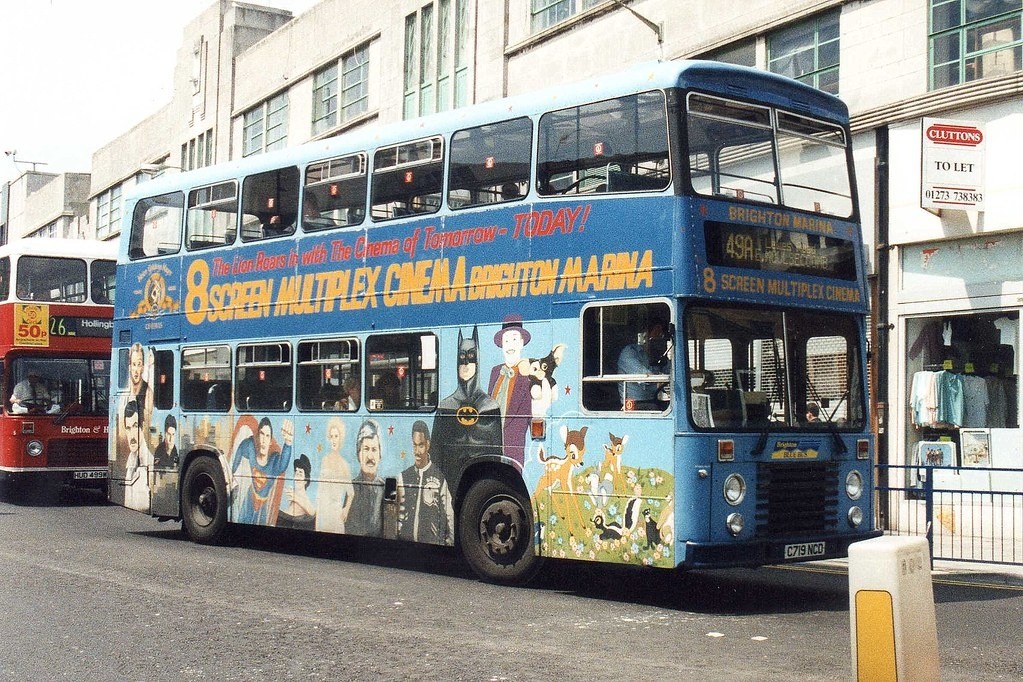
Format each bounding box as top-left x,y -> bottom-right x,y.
928,363 -> 1007,377
926,432 -> 946,442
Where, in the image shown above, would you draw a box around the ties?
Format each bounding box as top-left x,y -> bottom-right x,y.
31,385 -> 36,402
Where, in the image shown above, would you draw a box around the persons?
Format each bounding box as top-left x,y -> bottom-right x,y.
17,284 -> 30,298
10,365 -> 52,414
164,361 -> 201,412
806,403 -> 822,422
207,369 -> 231,412
91,282 -> 112,304
372,373 -> 401,410
336,376 -> 360,412
925,448 -> 943,466
263,349 -> 293,408
501,182 -> 520,200
539,183 -> 557,195
618,315 -> 715,411
282,191 -> 337,233
258,176 -> 297,234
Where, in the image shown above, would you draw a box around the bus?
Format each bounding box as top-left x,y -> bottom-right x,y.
107,60 -> 878,585
1,238 -> 125,486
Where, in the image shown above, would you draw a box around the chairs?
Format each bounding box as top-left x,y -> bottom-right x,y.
603,340 -> 623,404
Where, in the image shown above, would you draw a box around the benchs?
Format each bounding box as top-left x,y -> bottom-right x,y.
181,380 -> 338,411
158,163 -> 668,255
